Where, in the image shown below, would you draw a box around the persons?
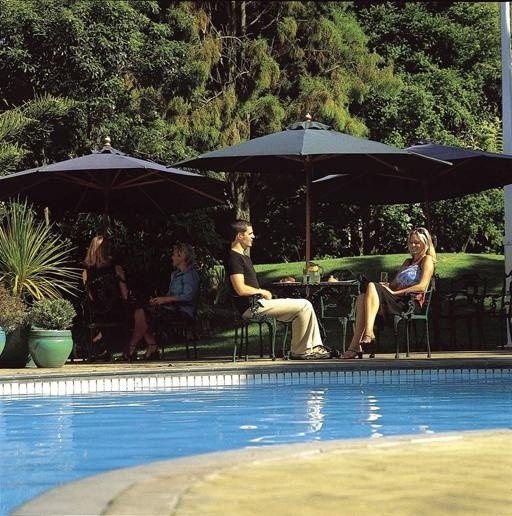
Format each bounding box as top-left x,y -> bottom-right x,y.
83,236 -> 161,362
225,220 -> 332,359
124,242 -> 203,362
338,227 -> 437,360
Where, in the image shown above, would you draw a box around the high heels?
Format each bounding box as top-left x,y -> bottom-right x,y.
338,335 -> 376,359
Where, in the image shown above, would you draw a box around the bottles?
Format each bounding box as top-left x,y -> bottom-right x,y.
303,267 -> 308,284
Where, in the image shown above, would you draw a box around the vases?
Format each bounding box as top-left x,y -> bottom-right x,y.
26,327 -> 75,367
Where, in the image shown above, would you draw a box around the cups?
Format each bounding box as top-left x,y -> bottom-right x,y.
380,272 -> 388,283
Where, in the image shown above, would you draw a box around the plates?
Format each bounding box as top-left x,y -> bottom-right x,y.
281,280 -> 300,283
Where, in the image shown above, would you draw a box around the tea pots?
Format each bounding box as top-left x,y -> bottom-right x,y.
308,262 -> 325,285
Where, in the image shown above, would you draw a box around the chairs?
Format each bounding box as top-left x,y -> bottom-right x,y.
78,265 -> 206,362
232,270 -> 512,361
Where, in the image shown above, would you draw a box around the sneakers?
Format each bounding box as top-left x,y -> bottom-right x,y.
291,346 -> 332,360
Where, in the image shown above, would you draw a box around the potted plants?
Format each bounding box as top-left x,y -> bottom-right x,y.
0,282 -> 27,356
0,192 -> 84,369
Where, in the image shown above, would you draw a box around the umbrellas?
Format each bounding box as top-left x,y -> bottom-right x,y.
166,114 -> 456,279
309,137 -> 512,254
1,137 -> 228,237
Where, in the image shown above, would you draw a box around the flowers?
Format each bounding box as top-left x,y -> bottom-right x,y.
28,299 -> 76,329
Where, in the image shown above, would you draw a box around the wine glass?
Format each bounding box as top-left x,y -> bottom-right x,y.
147,286 -> 158,302
